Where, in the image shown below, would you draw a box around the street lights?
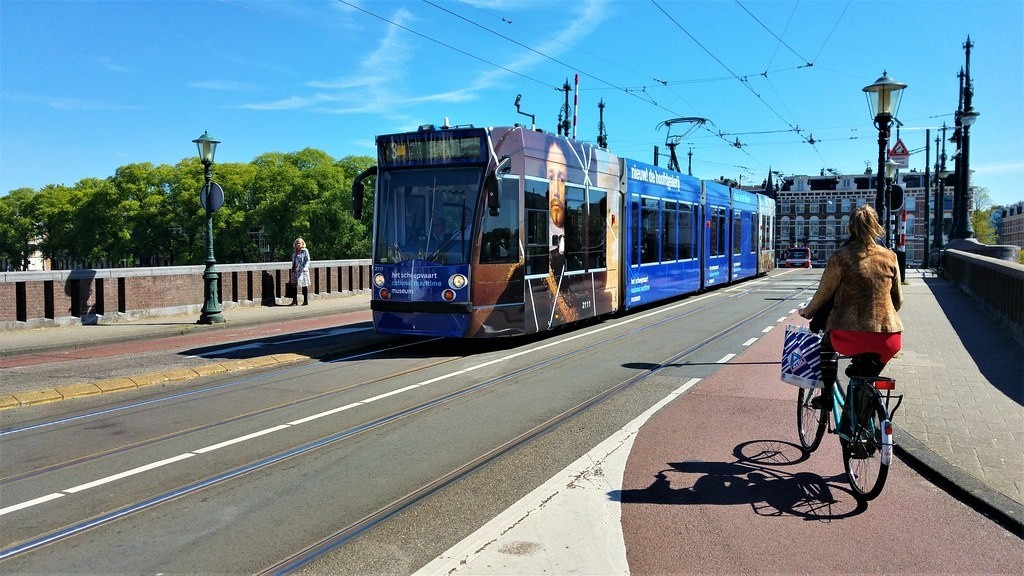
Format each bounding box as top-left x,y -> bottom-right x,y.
930,161 -> 949,269
950,103 -> 975,239
884,156 -> 902,249
861,66 -> 908,245
191,129 -> 227,324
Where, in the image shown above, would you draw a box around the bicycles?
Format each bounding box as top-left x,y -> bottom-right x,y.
796,297 -> 904,501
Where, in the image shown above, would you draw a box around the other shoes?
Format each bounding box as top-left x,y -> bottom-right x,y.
289,300 -> 297,306
811,395 -> 834,411
301,300 -> 308,306
849,443 -> 876,455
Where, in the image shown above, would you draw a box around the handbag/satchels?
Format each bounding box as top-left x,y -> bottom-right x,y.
781,323 -> 826,388
284,279 -> 294,298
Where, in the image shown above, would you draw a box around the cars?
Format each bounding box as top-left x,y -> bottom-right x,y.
811,259 -> 828,268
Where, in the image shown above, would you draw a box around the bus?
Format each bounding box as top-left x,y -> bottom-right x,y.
783,246 -> 814,268
352,117 -> 777,339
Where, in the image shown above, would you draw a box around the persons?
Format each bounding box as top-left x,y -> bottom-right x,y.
452,207 -> 473,240
430,218 -> 451,240
289,238 -> 311,306
803,243 -> 811,268
532,133 -> 581,292
798,204 -> 905,455
